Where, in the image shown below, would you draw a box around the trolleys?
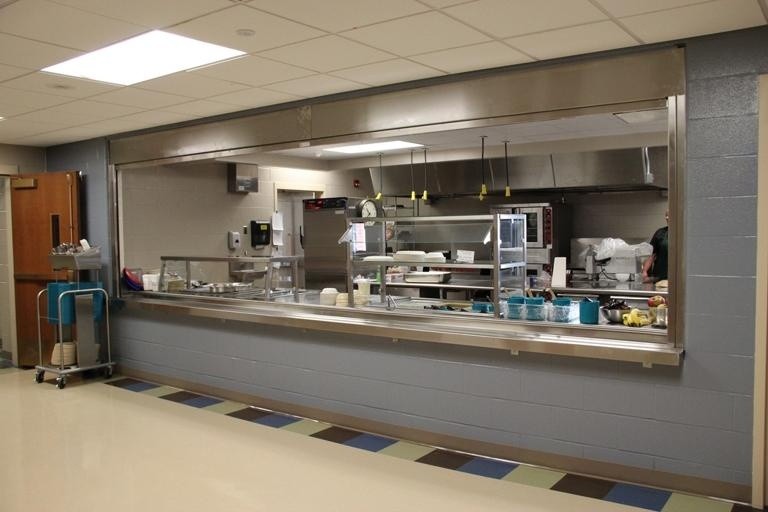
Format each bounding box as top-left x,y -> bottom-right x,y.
33,286 -> 117,389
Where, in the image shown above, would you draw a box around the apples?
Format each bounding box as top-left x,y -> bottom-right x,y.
647,295 -> 668,308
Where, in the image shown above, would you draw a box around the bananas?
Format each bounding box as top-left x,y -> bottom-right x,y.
622,308 -> 656,327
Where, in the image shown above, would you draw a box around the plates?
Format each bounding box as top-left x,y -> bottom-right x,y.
140,272 -> 185,293
51,341 -> 102,366
320,286 -> 359,306
361,250 -> 446,265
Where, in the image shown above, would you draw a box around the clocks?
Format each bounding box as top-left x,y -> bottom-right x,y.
358,199 -> 379,227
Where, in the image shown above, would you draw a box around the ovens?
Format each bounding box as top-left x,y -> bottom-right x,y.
490,200 -> 570,276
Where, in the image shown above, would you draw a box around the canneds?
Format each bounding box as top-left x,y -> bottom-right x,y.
530,276 -> 537,288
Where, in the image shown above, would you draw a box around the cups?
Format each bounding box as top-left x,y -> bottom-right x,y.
652,307 -> 668,327
579,299 -> 601,325
471,295 -> 571,325
356,278 -> 370,306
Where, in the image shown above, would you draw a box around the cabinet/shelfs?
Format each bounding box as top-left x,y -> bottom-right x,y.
345,214 -> 529,319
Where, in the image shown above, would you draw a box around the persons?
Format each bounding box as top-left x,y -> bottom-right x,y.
640,207 -> 669,284
386,222 -> 394,240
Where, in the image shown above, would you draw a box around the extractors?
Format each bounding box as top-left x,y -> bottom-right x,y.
370,147 -> 668,200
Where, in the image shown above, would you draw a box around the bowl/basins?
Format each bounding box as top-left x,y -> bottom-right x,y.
600,305 -> 634,324
